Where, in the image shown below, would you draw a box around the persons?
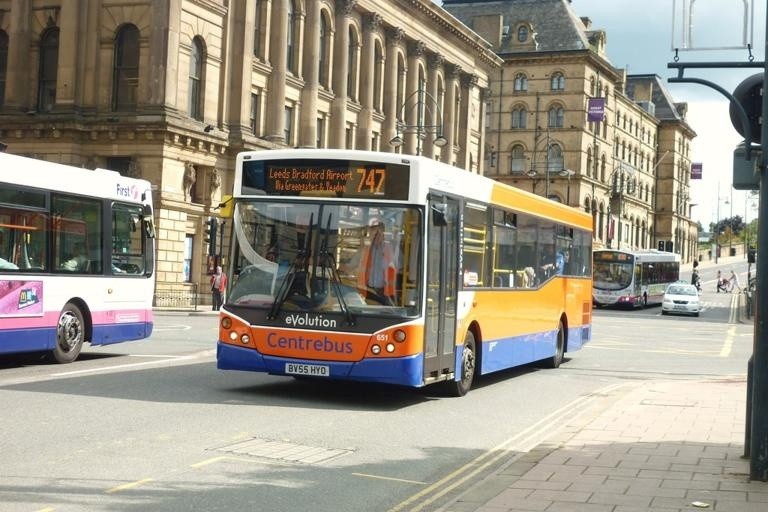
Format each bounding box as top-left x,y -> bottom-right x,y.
691,268 -> 704,295
0,231 -> 20,271
62,246 -> 88,273
727,270 -> 742,295
340,229 -> 397,306
538,239 -> 565,283
209,266 -> 228,311
716,270 -> 726,293
695,269 -> 702,291
693,257 -> 701,274
111,262 -> 127,274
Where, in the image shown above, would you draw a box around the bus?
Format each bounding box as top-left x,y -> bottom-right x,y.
205,145 -> 596,397
0,143 -> 160,366
585,245 -> 681,311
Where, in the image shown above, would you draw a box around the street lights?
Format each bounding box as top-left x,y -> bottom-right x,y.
601,159 -> 638,253
660,0 -> 766,486
384,87 -> 449,161
526,130 -> 570,202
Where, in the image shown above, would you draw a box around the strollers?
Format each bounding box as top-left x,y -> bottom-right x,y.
715,279 -> 729,293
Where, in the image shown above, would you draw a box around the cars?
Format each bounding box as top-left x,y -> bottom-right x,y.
659,281 -> 704,317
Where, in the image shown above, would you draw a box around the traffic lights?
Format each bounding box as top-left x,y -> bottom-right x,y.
748,247 -> 754,263
658,240 -> 663,250
730,247 -> 735,256
